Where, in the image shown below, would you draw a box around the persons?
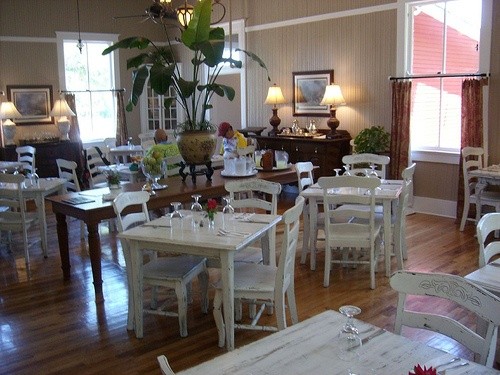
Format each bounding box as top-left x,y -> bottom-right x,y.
217,122 -> 246,155
153,129 -> 172,146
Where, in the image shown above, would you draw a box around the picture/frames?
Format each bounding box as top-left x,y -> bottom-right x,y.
6,83 -> 54,128
291,69 -> 335,117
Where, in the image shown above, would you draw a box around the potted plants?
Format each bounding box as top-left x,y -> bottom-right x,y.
100,0 -> 271,174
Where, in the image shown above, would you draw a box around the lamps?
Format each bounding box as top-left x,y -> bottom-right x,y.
264,84 -> 285,135
0,101 -> 23,147
319,82 -> 345,139
177,0 -> 194,28
49,96 -> 77,142
160,0 -> 172,3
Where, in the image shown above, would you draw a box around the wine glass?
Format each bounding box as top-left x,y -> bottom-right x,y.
170,202 -> 184,228
223,197 -> 235,221
190,194 -> 202,218
13,167 -> 27,190
332,168 -> 342,194
357,166 -> 379,195
342,165 -> 351,177
339,305 -> 362,362
0,168 -> 8,188
30,168 -> 39,186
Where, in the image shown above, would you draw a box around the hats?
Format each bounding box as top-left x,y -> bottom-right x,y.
217,121 -> 230,136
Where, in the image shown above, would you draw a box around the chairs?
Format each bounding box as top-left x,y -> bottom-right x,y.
294,160 -> 348,270
199,172 -> 279,318
15,145 -> 39,214
388,269 -> 500,369
213,195 -> 306,348
352,161 -> 419,271
56,159 -> 117,241
0,172 -> 43,266
111,189 -> 206,344
315,174 -> 383,292
341,152 -> 391,253
475,211 -> 500,268
459,145 -> 500,237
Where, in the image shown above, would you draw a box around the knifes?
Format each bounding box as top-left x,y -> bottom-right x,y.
239,221 -> 269,224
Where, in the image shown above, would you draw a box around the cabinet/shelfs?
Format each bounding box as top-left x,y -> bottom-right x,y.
254,129 -> 352,184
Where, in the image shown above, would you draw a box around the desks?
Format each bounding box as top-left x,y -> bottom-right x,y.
457,257 -> 500,365
114,204 -> 283,352
468,163 -> 500,235
0,139 -> 90,192
43,158 -> 323,306
0,160 -> 28,173
167,306 -> 500,375
0,177 -> 69,259
301,176 -> 402,276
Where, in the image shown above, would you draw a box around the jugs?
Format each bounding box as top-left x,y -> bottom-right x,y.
292,120 -> 300,134
256,151 -> 289,171
305,121 -> 317,134
